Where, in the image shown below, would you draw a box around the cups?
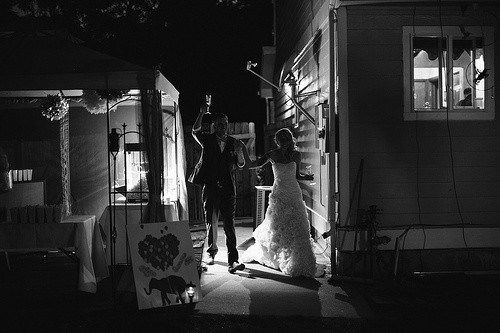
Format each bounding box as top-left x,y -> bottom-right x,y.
18,207 -> 27,223
54,207 -> 61,223
28,207 -> 35,223
45,207 -> 53,223
36,207 -> 44,223
10,208 -> 18,223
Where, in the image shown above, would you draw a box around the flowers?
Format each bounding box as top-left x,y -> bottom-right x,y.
82,90 -> 117,116
40,94 -> 69,121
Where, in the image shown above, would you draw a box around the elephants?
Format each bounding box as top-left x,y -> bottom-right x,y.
144,275 -> 187,306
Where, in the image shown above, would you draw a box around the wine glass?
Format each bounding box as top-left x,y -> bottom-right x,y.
205,95 -> 211,114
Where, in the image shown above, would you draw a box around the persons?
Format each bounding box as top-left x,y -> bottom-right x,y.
236,128 -> 319,279
455,87 -> 471,106
186,102 -> 247,274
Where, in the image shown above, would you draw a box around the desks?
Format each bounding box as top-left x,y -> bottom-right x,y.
40,213 -> 110,294
98,203 -> 172,267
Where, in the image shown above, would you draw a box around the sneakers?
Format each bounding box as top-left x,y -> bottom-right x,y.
228,261 -> 245,273
205,244 -> 218,265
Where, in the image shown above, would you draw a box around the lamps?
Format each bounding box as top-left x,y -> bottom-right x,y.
7,97 -> 38,105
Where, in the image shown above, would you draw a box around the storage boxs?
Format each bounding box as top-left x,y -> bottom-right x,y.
0,247 -> 80,308
